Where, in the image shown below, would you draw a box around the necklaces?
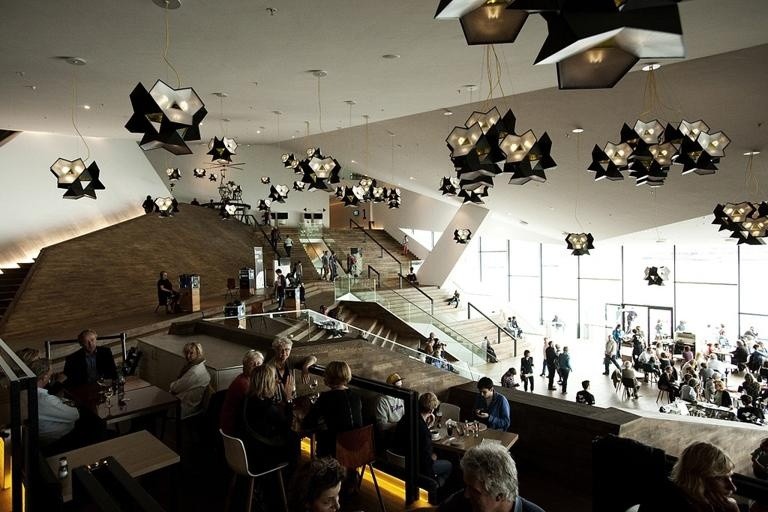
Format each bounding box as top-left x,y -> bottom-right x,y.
667,441 -> 742,512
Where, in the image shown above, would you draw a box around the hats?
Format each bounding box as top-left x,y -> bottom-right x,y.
386,373 -> 406,384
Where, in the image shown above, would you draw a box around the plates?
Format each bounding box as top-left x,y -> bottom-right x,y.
465,422 -> 487,431
431,435 -> 441,441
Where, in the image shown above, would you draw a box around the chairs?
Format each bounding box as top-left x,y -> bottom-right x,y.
609,340 -> 767,428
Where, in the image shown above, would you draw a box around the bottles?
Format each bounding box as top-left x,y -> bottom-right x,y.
96,375 -> 126,407
58,457 -> 69,480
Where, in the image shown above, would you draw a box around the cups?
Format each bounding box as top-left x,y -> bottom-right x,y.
430,429 -> 439,439
436,411 -> 442,423
446,420 -> 479,439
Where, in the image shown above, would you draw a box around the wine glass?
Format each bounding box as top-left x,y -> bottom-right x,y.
308,376 -> 320,405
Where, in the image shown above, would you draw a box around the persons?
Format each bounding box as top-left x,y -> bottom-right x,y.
275,260 -> 307,310
129,338 -> 208,437
62,330 -> 118,379
155,268 -> 179,311
303,360 -> 366,496
375,373 -> 405,450
319,305 -> 329,315
394,391 -> 454,482
29,355 -> 118,447
403,235 -> 409,255
142,194 -> 156,213
246,363 -> 300,511
408,267 -> 419,285
423,289 -> 768,433
437,441 -> 545,511
288,456 -> 346,512
191,197 -> 283,250
284,236 -> 294,256
219,336 -> 297,438
346,253 -> 359,278
322,250 -> 339,280
357,331 -> 368,341
10,347 -> 69,395
